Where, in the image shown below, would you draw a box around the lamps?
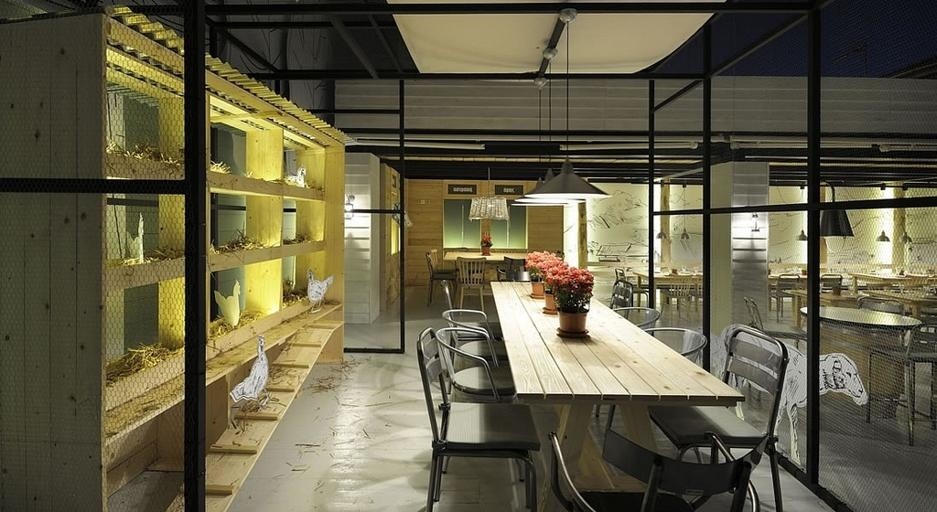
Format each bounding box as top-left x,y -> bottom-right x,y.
512,49 -> 587,205
899,184 -> 913,243
521,7 -> 612,200
468,164 -> 510,222
657,180 -> 667,239
751,213 -> 759,232
796,184 -> 807,241
680,184 -> 689,240
875,181 -> 891,242
344,193 -> 354,219
820,180 -> 855,252
508,73 -> 573,208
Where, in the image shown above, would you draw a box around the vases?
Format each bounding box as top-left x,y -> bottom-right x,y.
556,307 -> 590,340
542,291 -> 560,314
530,283 -> 547,298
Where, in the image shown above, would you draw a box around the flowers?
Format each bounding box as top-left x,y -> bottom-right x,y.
526,250 -> 561,280
538,260 -> 569,290
547,268 -> 596,306
479,231 -> 493,248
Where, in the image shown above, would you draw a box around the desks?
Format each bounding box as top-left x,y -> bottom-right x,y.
489,280 -> 746,511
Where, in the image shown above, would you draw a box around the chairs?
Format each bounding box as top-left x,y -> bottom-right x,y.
609,262 -> 937,448
649,325 -> 785,512
546,430 -> 775,511
433,326 -> 530,480
419,325 -> 537,507
594,305 -> 660,430
442,308 -> 516,396
444,283 -> 504,364
424,246 -> 526,311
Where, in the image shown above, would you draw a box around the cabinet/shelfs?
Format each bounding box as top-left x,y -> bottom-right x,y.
0,11 -> 345,511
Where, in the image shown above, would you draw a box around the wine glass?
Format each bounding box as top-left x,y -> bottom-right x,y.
657,263 -> 703,277
769,266 -> 803,277
